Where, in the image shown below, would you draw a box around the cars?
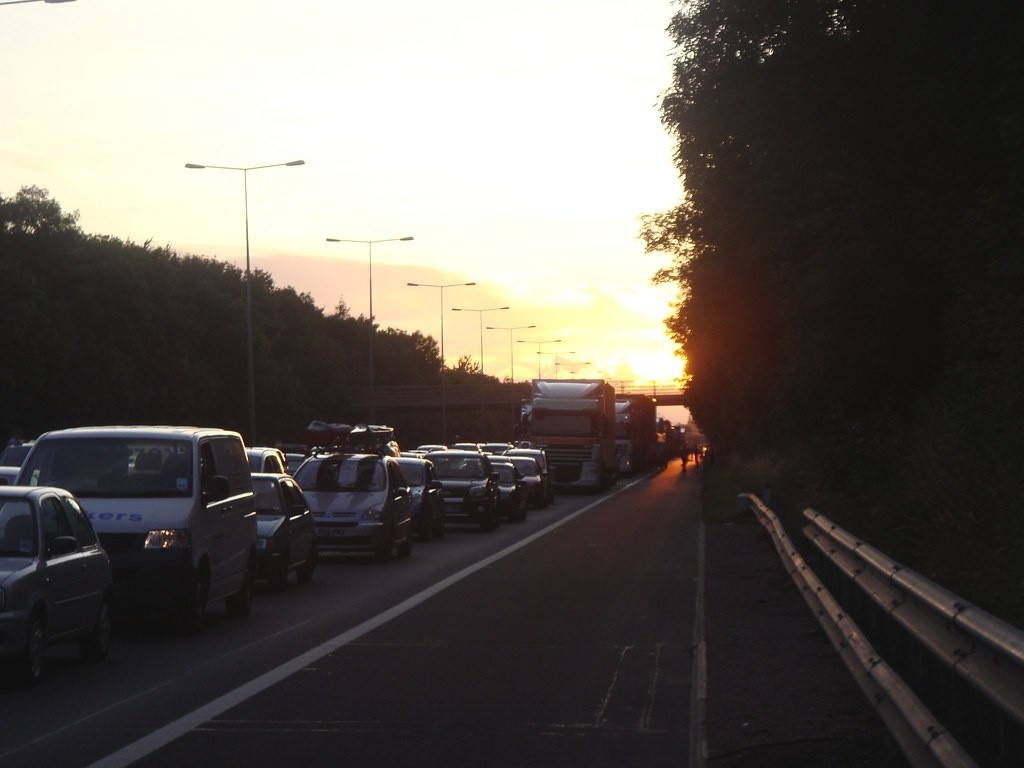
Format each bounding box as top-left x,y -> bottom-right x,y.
1,438 -> 46,489
283,452 -> 305,475
392,442 -> 554,543
0,484 -> 113,664
246,471 -> 318,599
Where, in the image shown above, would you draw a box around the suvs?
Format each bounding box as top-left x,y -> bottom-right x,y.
290,419 -> 413,566
246,447 -> 287,479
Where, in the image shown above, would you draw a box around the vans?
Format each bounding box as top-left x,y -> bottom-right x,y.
0,425 -> 260,642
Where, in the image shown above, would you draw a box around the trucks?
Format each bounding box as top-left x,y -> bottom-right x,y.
521,379 -> 617,497
612,391 -> 685,477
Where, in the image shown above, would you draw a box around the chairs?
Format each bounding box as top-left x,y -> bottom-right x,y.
122,453 -> 177,492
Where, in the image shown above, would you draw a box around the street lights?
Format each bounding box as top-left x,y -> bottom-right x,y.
182,159 -> 306,446
517,338 -> 564,381
451,306 -> 509,446
486,324 -> 536,384
535,349 -> 591,380
325,235 -> 416,423
408,280 -> 477,447
